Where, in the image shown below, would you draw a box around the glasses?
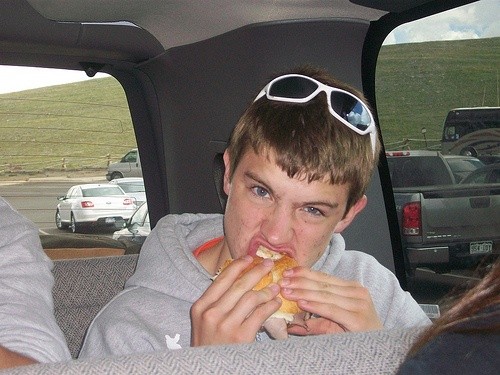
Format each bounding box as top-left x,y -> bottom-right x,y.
251,73 -> 378,161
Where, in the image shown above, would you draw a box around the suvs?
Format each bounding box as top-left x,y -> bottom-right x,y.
385,150 -> 500,280
105,147 -> 148,180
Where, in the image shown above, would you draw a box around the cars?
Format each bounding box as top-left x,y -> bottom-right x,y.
56,176 -> 152,238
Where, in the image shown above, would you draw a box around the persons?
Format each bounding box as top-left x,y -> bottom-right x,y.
76,73 -> 434,358
0,196 -> 72,371
393,257 -> 500,375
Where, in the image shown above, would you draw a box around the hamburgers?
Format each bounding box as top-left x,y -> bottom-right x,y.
212,256 -> 312,341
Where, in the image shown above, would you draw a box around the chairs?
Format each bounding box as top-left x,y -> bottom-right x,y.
51,254 -> 139,362
0,324 -> 433,375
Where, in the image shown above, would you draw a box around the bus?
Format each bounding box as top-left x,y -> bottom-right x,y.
442,106 -> 500,159
